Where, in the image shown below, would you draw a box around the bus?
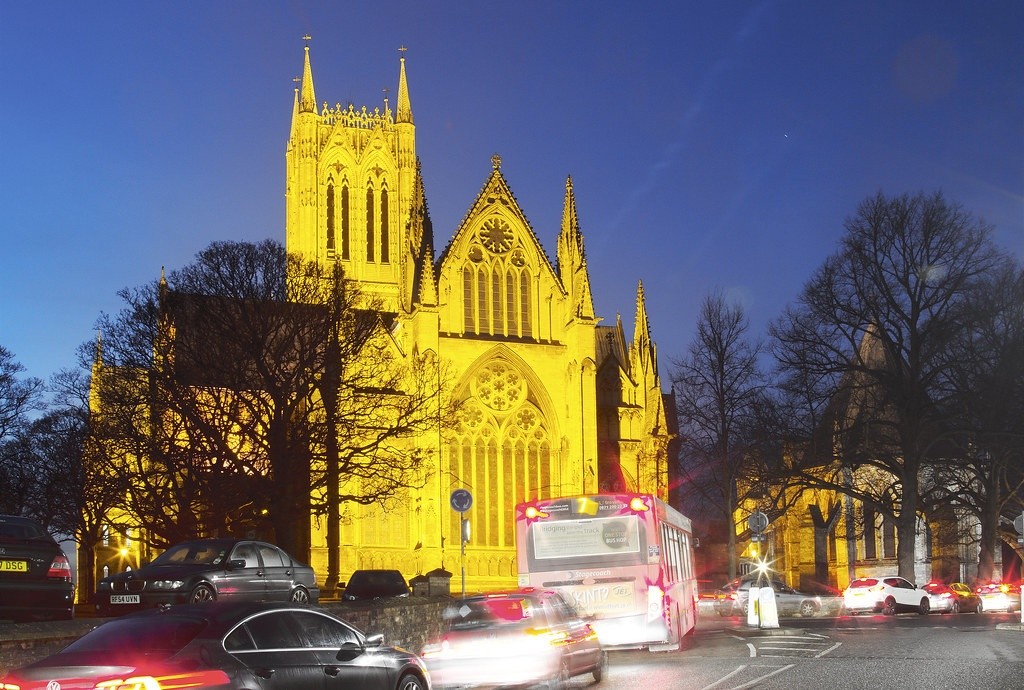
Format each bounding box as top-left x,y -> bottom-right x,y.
515,493 -> 700,653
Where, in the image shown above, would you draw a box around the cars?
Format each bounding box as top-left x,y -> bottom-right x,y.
975,584 -> 1021,612
713,580 -> 823,617
94,539 -> 318,615
0,607 -> 432,690
337,570 -> 415,603
948,584 -> 983,614
421,588 -> 607,689
920,583 -> 960,614
0,515 -> 74,618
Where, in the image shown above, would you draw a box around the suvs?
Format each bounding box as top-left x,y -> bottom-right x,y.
844,576 -> 930,615
